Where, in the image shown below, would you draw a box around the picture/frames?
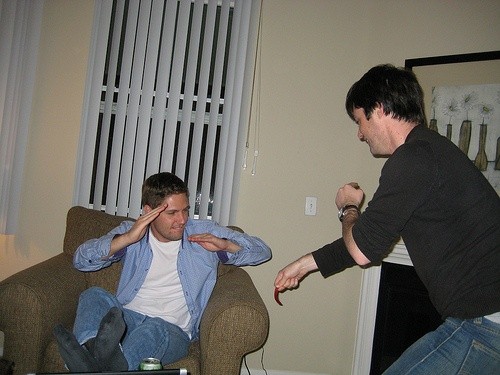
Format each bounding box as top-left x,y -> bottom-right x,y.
405,51 -> 500,199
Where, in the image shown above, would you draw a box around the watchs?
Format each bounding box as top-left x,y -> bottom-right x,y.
338,204 -> 361,223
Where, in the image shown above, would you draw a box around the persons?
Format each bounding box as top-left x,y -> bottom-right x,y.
275,64 -> 500,375
53,172 -> 273,374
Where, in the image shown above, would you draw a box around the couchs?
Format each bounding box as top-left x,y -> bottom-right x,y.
0,206 -> 270,375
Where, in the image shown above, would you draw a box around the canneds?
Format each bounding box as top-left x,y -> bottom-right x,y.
139,357 -> 162,370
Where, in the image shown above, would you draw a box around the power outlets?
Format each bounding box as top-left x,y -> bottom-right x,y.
305,197 -> 317,216
